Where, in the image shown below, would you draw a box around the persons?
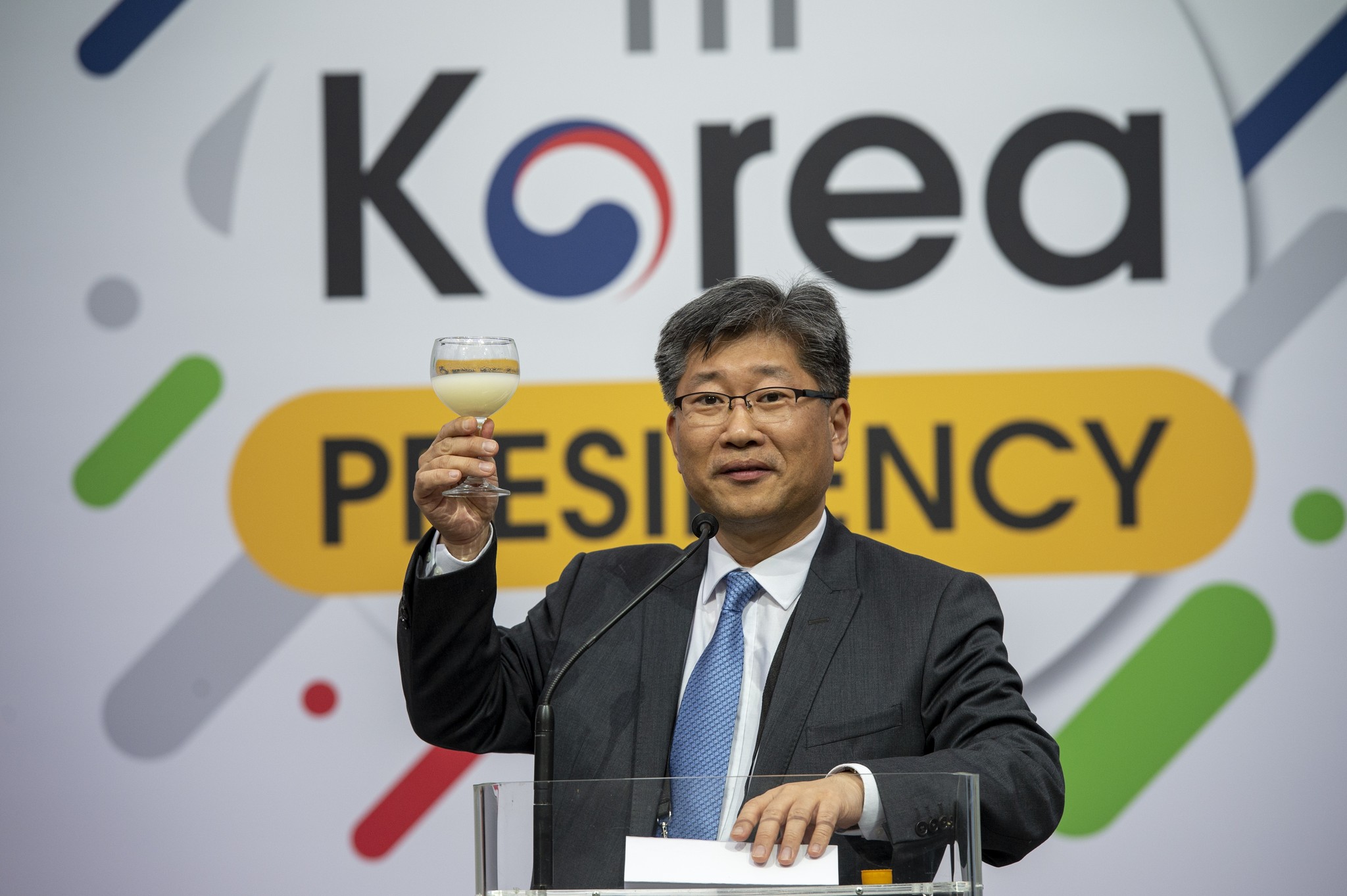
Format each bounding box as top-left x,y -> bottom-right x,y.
397,277 -> 1065,890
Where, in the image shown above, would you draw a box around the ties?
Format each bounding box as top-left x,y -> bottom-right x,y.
654,570 -> 764,840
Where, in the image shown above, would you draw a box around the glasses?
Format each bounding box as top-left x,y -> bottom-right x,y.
673,386 -> 838,423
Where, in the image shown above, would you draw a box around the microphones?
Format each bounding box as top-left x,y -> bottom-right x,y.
530,512 -> 720,882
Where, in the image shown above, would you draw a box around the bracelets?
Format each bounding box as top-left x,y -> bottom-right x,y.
834,766 -> 860,829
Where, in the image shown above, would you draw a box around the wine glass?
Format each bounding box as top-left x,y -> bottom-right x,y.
430,337 -> 520,497
